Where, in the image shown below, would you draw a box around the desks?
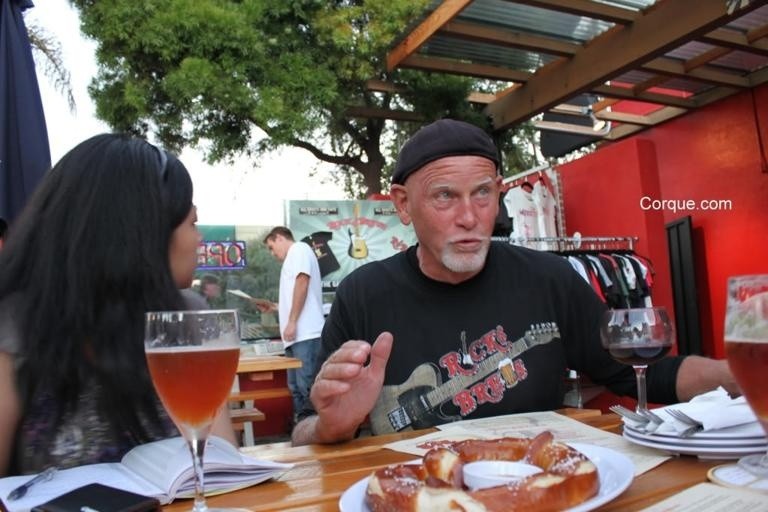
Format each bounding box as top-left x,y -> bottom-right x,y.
230,356 -> 303,392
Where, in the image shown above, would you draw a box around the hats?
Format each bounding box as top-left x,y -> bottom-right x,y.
391,119 -> 501,185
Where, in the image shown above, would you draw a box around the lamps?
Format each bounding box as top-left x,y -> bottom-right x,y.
584,104 -> 605,131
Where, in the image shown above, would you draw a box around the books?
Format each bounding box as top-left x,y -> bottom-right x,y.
0,426 -> 299,512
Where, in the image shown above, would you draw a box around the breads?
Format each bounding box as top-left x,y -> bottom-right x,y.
365,432 -> 599,512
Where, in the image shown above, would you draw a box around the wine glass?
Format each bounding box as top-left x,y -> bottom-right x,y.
723,273 -> 768,477
599,306 -> 674,431
142,308 -> 255,512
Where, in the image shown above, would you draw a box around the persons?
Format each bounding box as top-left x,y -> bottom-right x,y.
288,117 -> 744,448
0,130 -> 239,479
250,226 -> 328,427
199,274 -> 224,309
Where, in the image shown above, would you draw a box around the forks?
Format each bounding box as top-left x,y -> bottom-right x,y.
608,404 -> 701,428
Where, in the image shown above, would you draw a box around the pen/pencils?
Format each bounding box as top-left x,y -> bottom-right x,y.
6,467 -> 58,502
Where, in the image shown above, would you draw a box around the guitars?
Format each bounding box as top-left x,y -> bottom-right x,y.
347,204 -> 369,260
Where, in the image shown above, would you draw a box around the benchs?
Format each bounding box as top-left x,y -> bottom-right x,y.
230,408 -> 265,446
229,388 -> 291,408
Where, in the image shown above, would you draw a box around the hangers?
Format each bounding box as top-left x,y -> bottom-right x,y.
501,171 -> 545,189
497,236 -> 618,252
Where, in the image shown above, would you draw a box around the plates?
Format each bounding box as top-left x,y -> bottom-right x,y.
337,443 -> 636,512
622,401 -> 768,461
